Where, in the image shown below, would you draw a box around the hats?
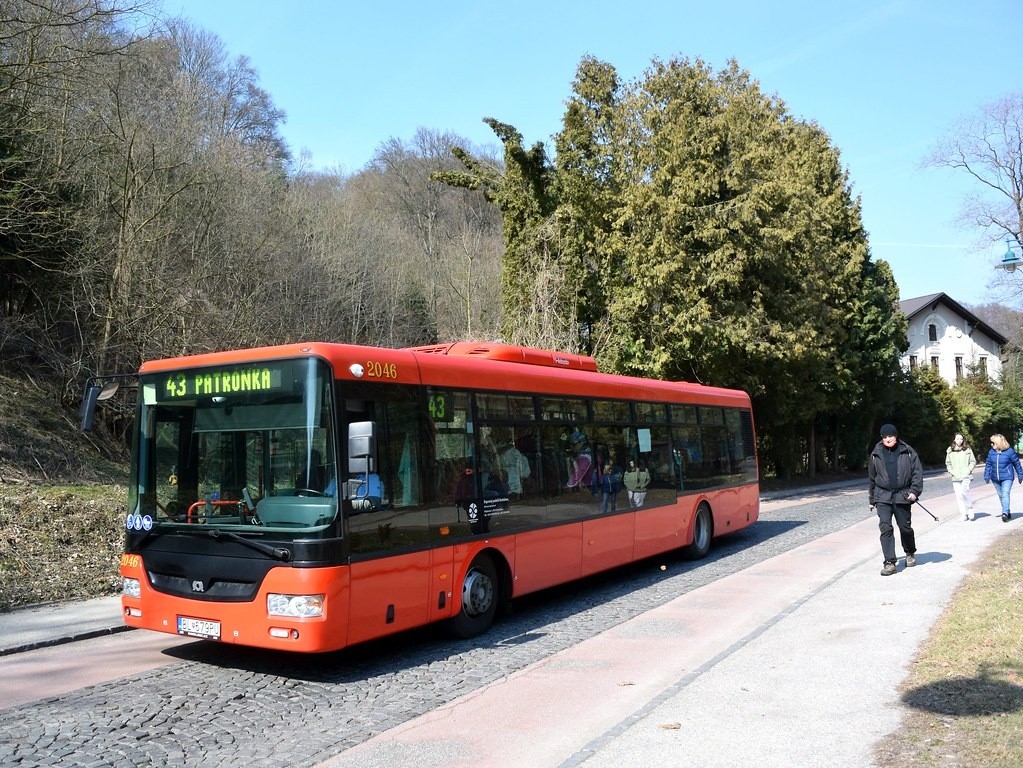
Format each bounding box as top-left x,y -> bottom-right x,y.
488,426 -> 513,443
464,461 -> 474,469
569,433 -> 586,443
880,424 -> 897,437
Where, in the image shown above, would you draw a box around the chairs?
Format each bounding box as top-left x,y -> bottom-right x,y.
434,440 -> 745,498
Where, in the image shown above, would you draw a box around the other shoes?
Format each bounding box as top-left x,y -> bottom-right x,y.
961,509 -> 974,522
1002,513 -> 1012,521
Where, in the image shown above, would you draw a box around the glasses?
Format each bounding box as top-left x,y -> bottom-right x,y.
571,440 -> 582,448
607,467 -> 613,471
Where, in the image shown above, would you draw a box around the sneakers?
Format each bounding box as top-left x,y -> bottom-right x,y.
881,562 -> 897,575
905,550 -> 915,567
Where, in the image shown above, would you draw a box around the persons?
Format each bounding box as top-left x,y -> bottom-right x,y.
867,424 -> 925,575
531,433 -> 568,497
623,460 -> 650,508
984,435 -> 1023,522
945,432 -> 977,521
453,460 -> 509,535
296,449 -> 329,496
322,456 -> 385,511
488,427 -> 531,497
565,434 -> 605,490
600,464 -> 622,513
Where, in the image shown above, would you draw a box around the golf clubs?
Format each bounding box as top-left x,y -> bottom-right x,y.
904,491 -> 940,521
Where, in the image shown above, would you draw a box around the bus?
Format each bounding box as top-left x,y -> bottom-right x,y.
77,341 -> 765,653
